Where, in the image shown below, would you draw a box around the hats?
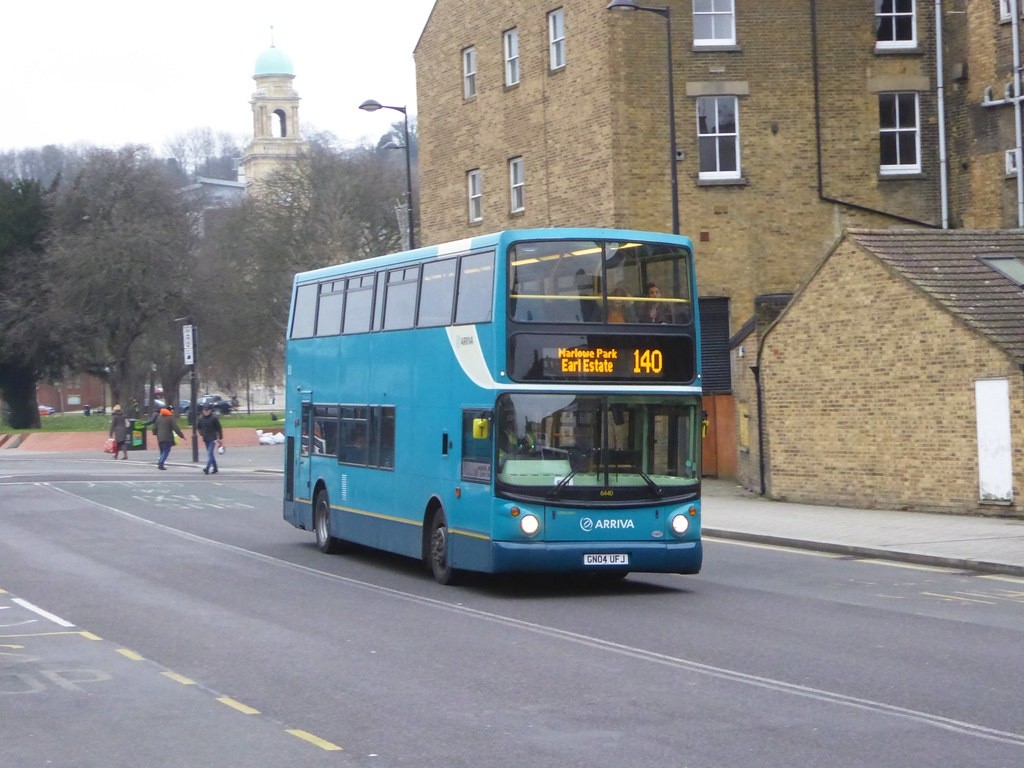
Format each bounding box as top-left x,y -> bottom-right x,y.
113,405 -> 121,411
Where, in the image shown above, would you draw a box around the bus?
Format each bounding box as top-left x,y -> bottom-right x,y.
283,224 -> 705,587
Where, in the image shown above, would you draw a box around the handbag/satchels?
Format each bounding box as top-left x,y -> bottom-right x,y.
103,438 -> 119,453
125,419 -> 131,428
214,439 -> 226,454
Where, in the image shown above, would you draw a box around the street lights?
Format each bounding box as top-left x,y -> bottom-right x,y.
357,99 -> 414,253
608,0 -> 685,323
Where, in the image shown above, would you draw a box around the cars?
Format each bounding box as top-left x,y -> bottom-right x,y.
29,389 -> 235,418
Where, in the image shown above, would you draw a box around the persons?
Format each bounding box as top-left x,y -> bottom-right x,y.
109,404 -> 129,461
639,283 -> 673,324
589,287 -> 638,324
197,405 -> 224,475
152,405 -> 187,470
498,412 -> 533,472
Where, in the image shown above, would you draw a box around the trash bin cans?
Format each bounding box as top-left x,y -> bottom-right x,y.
84,405 -> 90,416
123,418 -> 147,451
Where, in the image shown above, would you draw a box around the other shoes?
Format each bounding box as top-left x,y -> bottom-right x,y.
157,463 -> 167,470
203,468 -> 218,475
113,456 -> 128,460
